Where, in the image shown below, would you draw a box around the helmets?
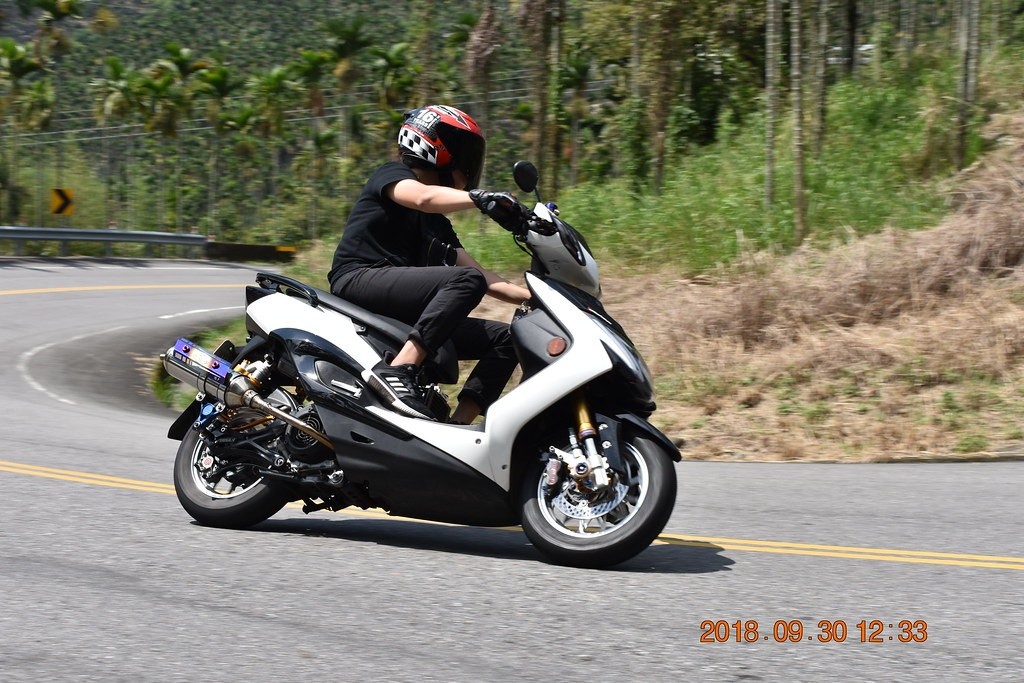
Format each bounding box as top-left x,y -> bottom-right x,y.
397,105 -> 485,190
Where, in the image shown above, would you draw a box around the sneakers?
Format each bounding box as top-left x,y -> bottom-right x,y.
360,350 -> 438,421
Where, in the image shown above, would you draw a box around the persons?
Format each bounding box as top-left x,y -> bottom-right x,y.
328,105 -> 533,425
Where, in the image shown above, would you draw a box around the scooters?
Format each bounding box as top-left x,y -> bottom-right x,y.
159,159 -> 682,569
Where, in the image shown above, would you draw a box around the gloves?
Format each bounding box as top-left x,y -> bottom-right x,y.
469,189 -> 532,220
